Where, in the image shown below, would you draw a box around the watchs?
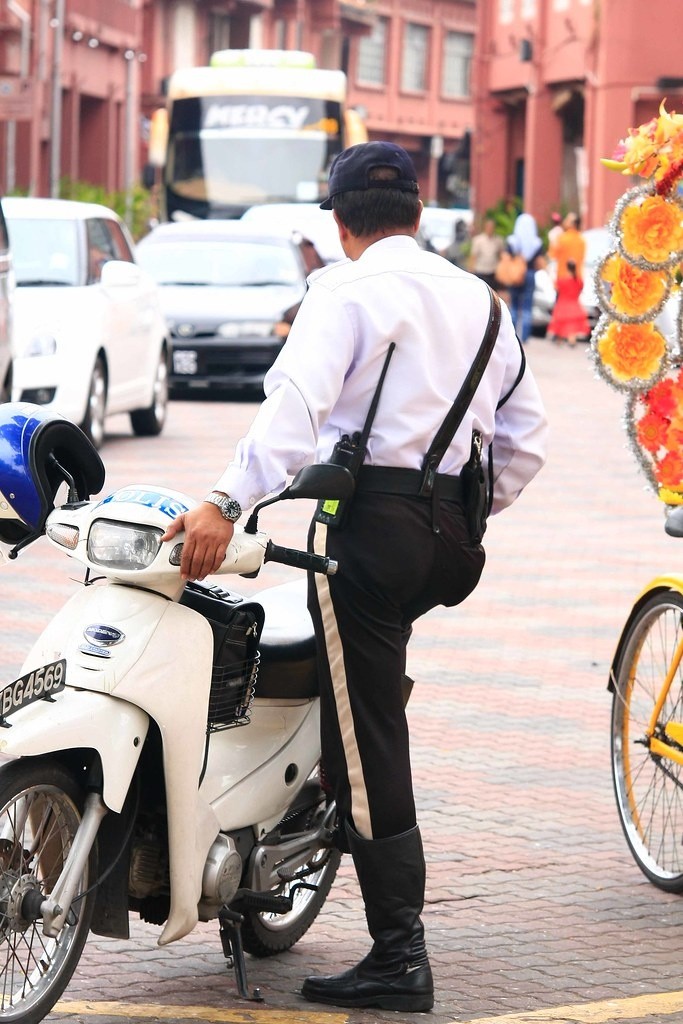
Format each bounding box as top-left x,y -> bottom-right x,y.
204,494 -> 242,523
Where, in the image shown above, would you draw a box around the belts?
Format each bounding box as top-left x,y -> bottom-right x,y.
346,464 -> 473,502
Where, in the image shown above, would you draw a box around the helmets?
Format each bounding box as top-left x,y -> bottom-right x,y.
0,399 -> 107,545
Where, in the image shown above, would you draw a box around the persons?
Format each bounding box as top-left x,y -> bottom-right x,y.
446,213 -> 590,347
161,143 -> 551,1012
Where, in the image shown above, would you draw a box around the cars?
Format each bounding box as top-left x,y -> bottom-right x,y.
241,203 -> 348,266
2,196 -> 176,448
604,503 -> 683,892
415,203 -> 470,258
132,219 -> 330,397
532,229 -> 617,341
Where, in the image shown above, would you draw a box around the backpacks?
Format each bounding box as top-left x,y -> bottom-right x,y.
495,250 -> 529,287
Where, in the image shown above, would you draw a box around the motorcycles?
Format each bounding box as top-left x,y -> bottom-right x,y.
0,400 -> 347,1024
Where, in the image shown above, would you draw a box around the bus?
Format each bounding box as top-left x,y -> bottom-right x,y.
147,45 -> 370,219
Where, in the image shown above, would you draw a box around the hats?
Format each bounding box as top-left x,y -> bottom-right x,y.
550,210 -> 561,222
319,141 -> 420,210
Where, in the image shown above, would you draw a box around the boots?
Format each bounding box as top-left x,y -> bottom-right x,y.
398,670 -> 415,721
302,816 -> 435,1013
